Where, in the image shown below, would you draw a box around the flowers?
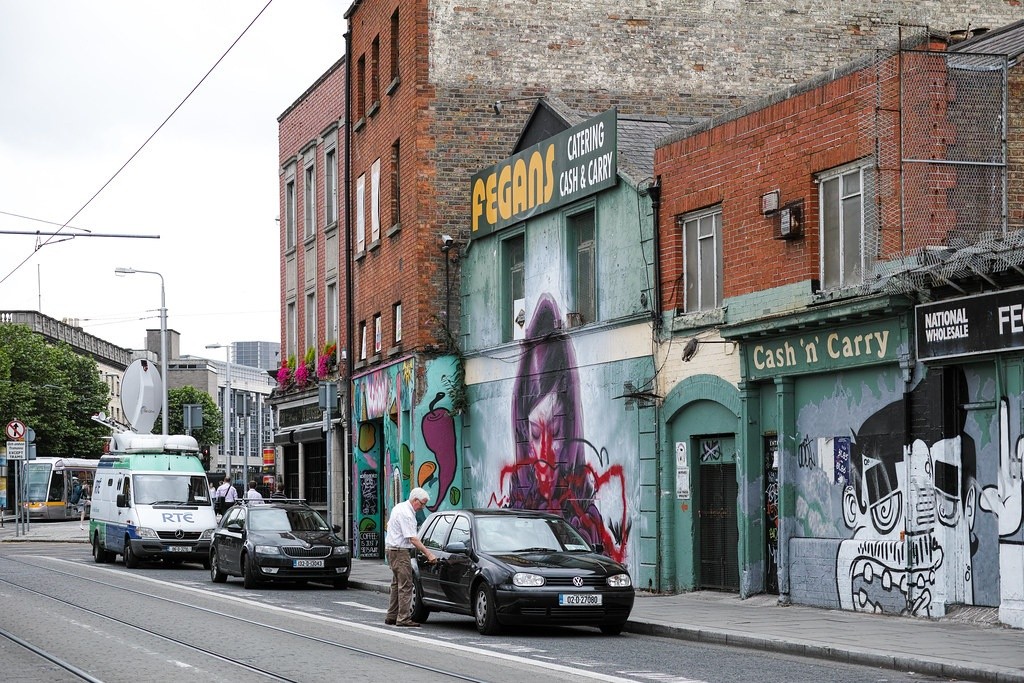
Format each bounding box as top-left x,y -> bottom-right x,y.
276,341 -> 336,388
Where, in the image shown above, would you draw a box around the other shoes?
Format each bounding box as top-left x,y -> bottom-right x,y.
396,621 -> 420,627
384,618 -> 396,624
79,525 -> 84,530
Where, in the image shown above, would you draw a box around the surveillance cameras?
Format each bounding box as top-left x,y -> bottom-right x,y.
442,235 -> 453,246
271,426 -> 275,431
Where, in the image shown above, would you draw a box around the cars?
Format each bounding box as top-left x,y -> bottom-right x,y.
210,503 -> 351,589
405,509 -> 634,635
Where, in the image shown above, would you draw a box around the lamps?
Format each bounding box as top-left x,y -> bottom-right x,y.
493,95 -> 542,114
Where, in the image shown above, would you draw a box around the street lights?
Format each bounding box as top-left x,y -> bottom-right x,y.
206,343 -> 230,482
115,266 -> 168,435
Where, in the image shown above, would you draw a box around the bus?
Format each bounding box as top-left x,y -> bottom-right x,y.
19,456 -> 100,522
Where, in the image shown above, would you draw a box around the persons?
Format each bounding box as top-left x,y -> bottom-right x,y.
70,482 -> 91,530
210,476 -> 288,518
385,487 -> 438,627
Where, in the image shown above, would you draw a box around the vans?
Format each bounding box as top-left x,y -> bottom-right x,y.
89,452 -> 216,570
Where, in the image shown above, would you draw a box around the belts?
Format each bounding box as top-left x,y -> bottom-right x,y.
391,548 -> 409,551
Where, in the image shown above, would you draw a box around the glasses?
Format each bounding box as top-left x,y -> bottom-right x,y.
418,499 -> 426,506
